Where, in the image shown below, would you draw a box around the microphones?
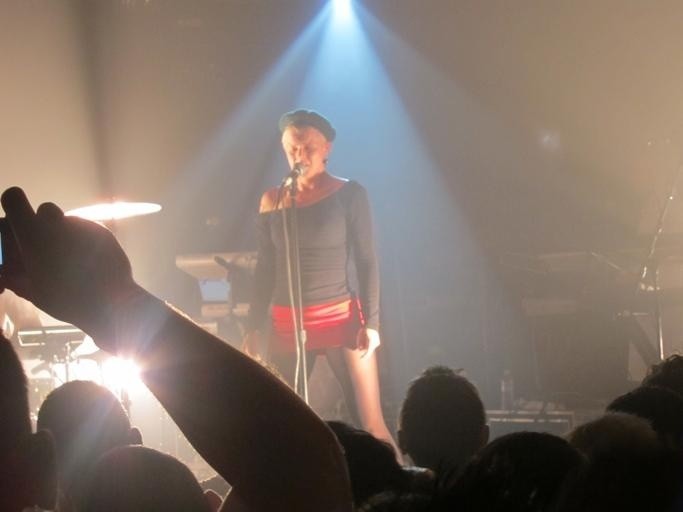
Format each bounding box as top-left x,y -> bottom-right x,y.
280,163 -> 308,190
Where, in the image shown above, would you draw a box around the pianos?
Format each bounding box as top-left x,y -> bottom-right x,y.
14,321 -> 219,357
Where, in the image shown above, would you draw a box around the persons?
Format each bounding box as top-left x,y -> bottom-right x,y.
0,186 -> 682,510
242,112 -> 407,470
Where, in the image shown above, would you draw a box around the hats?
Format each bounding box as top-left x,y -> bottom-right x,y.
279,110 -> 335,143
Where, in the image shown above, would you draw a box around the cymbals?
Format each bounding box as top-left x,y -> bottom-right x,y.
62,202 -> 162,221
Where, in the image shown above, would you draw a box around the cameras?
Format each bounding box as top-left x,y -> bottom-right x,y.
0,217 -> 19,287
